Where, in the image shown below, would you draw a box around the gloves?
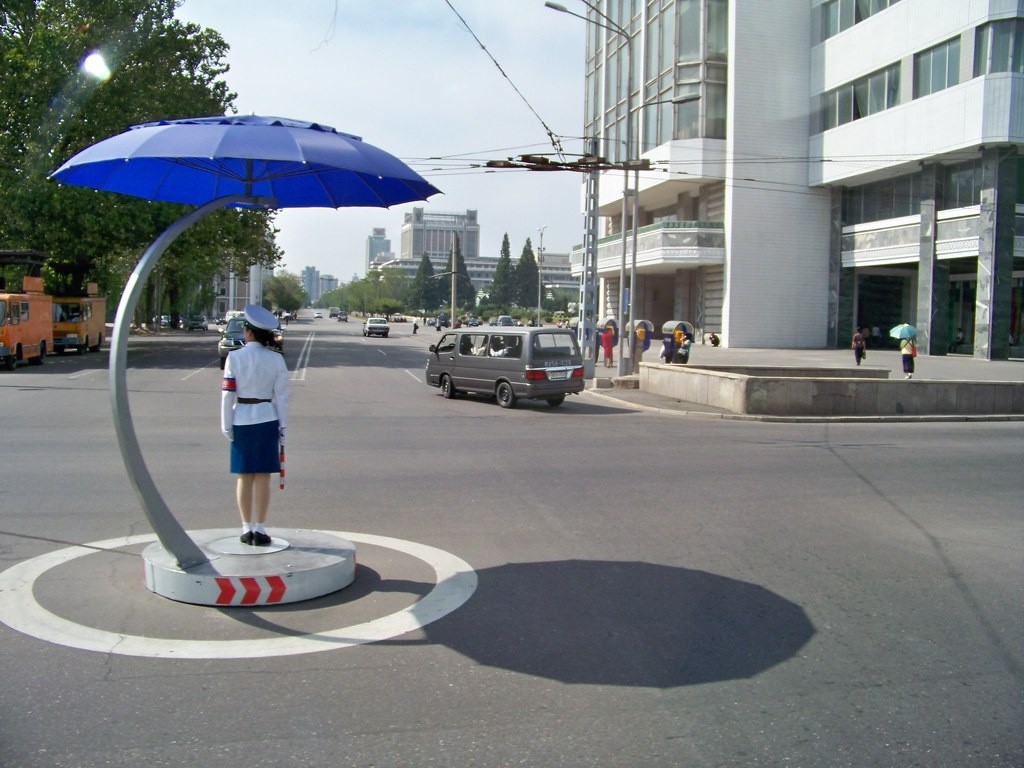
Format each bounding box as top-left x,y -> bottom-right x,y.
222,429 -> 234,441
279,428 -> 287,445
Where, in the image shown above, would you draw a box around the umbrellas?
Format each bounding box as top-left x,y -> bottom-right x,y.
46,113 -> 446,570
889,323 -> 917,339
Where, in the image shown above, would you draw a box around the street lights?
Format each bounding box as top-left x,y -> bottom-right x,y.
629,93 -> 701,372
537,225 -> 548,328
543,1 -> 635,376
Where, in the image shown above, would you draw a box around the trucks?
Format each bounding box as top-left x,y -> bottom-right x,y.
329,306 -> 340,318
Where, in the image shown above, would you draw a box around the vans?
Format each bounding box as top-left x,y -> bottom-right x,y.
425,327 -> 586,408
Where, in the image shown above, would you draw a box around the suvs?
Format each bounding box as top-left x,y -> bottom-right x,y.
218,316 -> 284,369
188,316 -> 208,331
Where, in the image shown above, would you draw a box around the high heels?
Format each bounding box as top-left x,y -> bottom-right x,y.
240,531 -> 254,545
254,532 -> 271,546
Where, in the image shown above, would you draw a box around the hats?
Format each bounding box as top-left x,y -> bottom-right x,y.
241,304 -> 279,333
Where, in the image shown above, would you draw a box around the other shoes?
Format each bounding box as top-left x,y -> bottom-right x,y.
905,373 -> 911,379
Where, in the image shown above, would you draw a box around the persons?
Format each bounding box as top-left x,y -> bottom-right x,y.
436,317 -> 441,331
900,338 -> 914,380
273,308 -> 300,325
517,319 -> 534,327
413,315 -> 418,334
862,322 -> 882,343
556,319 -> 570,329
454,319 -> 462,330
948,328 -> 964,353
489,336 -> 518,357
709,333 -> 720,347
221,304 -> 288,546
1009,333 -> 1015,346
681,333 -> 691,363
595,328 -> 613,367
852,327 -> 866,366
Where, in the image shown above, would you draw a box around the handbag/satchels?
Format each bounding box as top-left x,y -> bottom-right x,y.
678,347 -> 688,356
913,346 -> 916,357
863,352 -> 866,359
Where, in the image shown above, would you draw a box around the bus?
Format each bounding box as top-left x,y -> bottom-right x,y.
0,293 -> 54,371
51,298 -> 106,354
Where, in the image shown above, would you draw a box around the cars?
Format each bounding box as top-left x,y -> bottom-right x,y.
314,312 -> 322,318
152,315 -> 171,326
427,316 -> 524,327
363,318 -> 390,338
337,311 -> 348,322
